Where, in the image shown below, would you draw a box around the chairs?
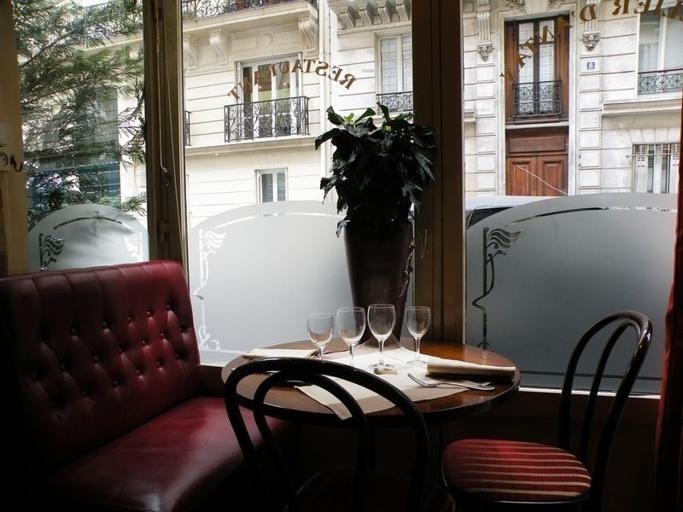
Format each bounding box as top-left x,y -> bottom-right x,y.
224,356 -> 442,511
441,309 -> 653,510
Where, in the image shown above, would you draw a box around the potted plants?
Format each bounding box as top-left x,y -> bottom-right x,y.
312,101 -> 440,343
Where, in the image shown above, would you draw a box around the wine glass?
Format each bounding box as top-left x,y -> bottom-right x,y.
307,305 -> 431,375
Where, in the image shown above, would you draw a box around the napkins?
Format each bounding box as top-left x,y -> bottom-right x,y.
426,356 -> 516,382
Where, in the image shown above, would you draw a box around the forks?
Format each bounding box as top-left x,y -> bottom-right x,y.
406,373 -> 495,392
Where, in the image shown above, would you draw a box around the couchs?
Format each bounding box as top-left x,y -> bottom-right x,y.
0,259 -> 295,512
